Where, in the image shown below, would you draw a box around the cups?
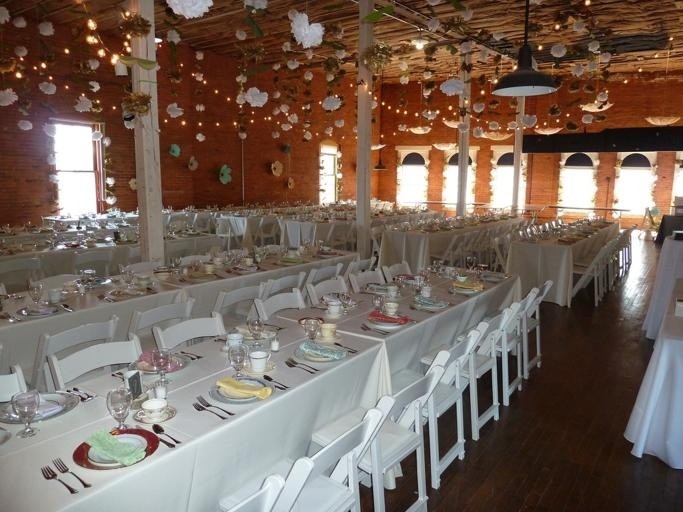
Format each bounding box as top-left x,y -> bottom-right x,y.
321,323 -> 338,338
203,262 -> 216,274
387,286 -> 400,299
384,302 -> 399,315
49,288 -> 66,304
414,276 -> 425,284
328,301 -> 340,315
243,256 -> 255,267
138,275 -> 155,288
456,275 -> 467,283
225,334 -> 245,348
249,350 -> 272,371
420,287 -> 432,298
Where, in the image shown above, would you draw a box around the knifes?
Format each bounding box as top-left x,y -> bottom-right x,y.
136,425 -> 176,449
334,342 -> 357,352
264,375 -> 291,390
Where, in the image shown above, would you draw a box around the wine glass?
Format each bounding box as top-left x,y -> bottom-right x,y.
228,342 -> 249,377
338,289 -> 353,315
106,388 -> 134,430
82,267 -> 97,291
465,256 -> 477,270
25,278 -> 45,302
151,347 -> 173,386
11,389 -> 41,440
371,294 -> 385,312
119,266 -> 135,288
248,318 -> 265,348
303,319 -> 320,340
50,233 -> 57,248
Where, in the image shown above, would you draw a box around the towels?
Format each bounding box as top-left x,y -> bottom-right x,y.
322,293 -> 356,308
319,250 -> 337,256
299,340 -> 347,361
139,351 -> 181,373
29,389 -> 62,418
415,294 -> 449,310
235,264 -> 256,272
280,257 -> 297,263
367,281 -> 399,297
368,310 -> 410,326
192,271 -> 208,280
235,325 -> 276,340
84,426 -> 146,467
453,279 -> 484,290
122,287 -> 144,296
32,305 -> 55,314
397,273 -> 415,281
481,271 -> 506,278
215,376 -> 272,399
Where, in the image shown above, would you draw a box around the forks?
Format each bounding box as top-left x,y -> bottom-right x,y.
196,395 -> 235,417
176,352 -> 199,362
40,464 -> 78,496
284,361 -> 317,376
66,389 -> 91,403
289,358 -> 320,373
52,458 -> 92,490
193,402 -> 228,421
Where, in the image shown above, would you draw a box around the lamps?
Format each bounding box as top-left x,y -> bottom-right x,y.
619,154 -> 651,168
401,152 -> 425,167
446,151 -> 472,166
373,63 -> 389,171
495,152 -> 523,167
490,2 -> 559,98
563,153 -> 593,168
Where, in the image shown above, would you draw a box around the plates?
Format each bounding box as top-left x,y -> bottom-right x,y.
234,322 -> 279,341
237,360 -> 277,378
298,341 -> 336,362
221,343 -> 248,353
73,428 -> 160,471
368,313 -> 408,329
87,433 -> 148,466
366,282 -> 401,299
134,405 -> 178,424
208,375 -> 275,405
16,304 -> 61,318
0,391 -> 80,425
320,292 -> 356,310
448,285 -> 478,295
180,254 -> 211,265
480,274 -> 505,282
411,298 -> 449,312
128,352 -> 191,375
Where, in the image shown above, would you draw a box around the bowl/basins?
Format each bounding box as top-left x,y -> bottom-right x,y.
298,317 -> 325,328
142,397 -> 168,420
153,270 -> 172,280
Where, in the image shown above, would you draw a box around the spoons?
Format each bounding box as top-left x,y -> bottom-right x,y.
152,425 -> 181,445
180,351 -> 204,360
73,388 -> 98,400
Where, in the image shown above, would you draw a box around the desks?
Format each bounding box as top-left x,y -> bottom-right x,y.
163,206 -> 216,231
278,212 -> 460,250
0,265 -> 521,512
0,243 -> 361,391
382,210 -> 523,275
382,213 -> 529,251
218,207 -> 358,248
508,219 -> 621,307
654,214 -> 683,245
0,232 -> 228,294
624,276 -> 683,469
641,234 -> 683,341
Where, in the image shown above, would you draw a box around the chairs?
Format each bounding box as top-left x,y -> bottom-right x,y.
303,276 -> 349,307
125,246 -> 141,266
420,307 -> 513,442
496,224 -> 503,234
347,267 -> 386,293
531,225 -> 538,235
506,232 -> 514,243
0,256 -> 48,286
311,365 -> 446,512
256,212 -> 281,248
457,231 -> 480,265
511,222 -> 519,230
151,310 -> 227,353
519,219 -> 528,230
225,473 -> 286,512
323,224 -> 349,250
46,332 -> 144,391
413,219 -> 425,231
492,237 -> 514,272
212,281 -> 266,315
348,221 -> 357,249
34,314 -> 119,394
486,227 -> 495,239
538,225 -> 544,234
429,234 -> 465,265
518,280 -> 554,380
402,221 -> 412,232
470,229 -> 489,264
216,218 -> 240,250
390,329 -> 481,489
300,261 -> 344,298
544,222 -> 554,232
517,230 -> 525,241
249,288 -> 309,320
342,256 -> 377,282
0,361 -> 30,402
571,223 -> 639,308
385,221 -> 400,231
551,220 -> 561,229
128,297 -> 194,335
528,217 -> 536,237
527,228 -> 534,237
504,222 -> 510,233
457,286 -> 540,408
636,206 -> 658,240
367,224 -> 384,257
557,219 -> 569,226
286,408 -> 384,512
68,247 -> 114,276
264,270 -> 306,297
381,260 -> 413,284
